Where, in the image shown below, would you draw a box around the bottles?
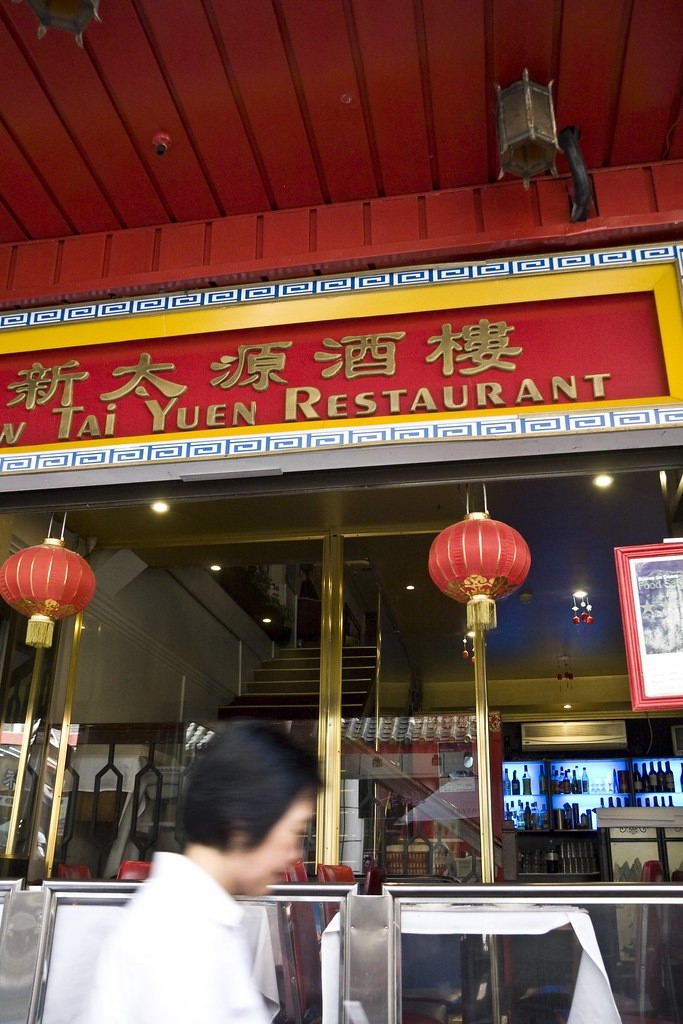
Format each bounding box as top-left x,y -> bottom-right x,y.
656,761 -> 667,792
572,770 -> 578,794
512,770 -> 520,795
633,763 -> 643,793
503,800 -> 518,829
539,765 -> 547,795
649,762 -> 659,792
551,765 -> 559,794
613,769 -> 631,793
642,763 -> 651,793
531,802 -> 549,830
523,765 -> 532,795
624,796 -> 674,808
665,761 -> 675,792
680,763 -> 683,793
516,800 -> 531,830
552,803 -> 592,830
559,766 -> 572,794
575,766 -> 582,794
503,769 -> 511,796
600,797 -> 622,808
518,840 -> 559,874
582,768 -> 589,794
559,842 -> 597,873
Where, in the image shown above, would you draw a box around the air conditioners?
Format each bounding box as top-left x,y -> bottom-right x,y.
522,721 -> 628,752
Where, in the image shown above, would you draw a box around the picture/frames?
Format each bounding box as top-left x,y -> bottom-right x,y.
615,540 -> 683,714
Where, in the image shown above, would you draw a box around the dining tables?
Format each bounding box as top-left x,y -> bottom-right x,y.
320,900 -> 623,1024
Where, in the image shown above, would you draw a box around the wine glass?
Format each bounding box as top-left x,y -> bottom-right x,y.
591,784 -> 613,794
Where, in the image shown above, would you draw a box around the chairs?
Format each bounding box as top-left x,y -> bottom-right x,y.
276,863 -> 465,1024
520,858 -> 663,1024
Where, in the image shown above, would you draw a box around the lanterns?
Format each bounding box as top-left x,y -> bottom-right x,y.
27,0 -> 103,51
0,538 -> 96,646
493,66 -> 560,187
428,512 -> 531,630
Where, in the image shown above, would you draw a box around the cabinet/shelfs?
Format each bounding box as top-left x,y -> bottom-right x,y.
549,758 -> 633,831
502,762 -> 551,830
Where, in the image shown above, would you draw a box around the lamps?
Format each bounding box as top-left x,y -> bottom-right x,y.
18,0 -> 102,52
574,590 -> 594,626
495,71 -> 565,189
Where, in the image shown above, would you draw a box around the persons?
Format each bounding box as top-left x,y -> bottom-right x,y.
78,723 -> 325,1024
